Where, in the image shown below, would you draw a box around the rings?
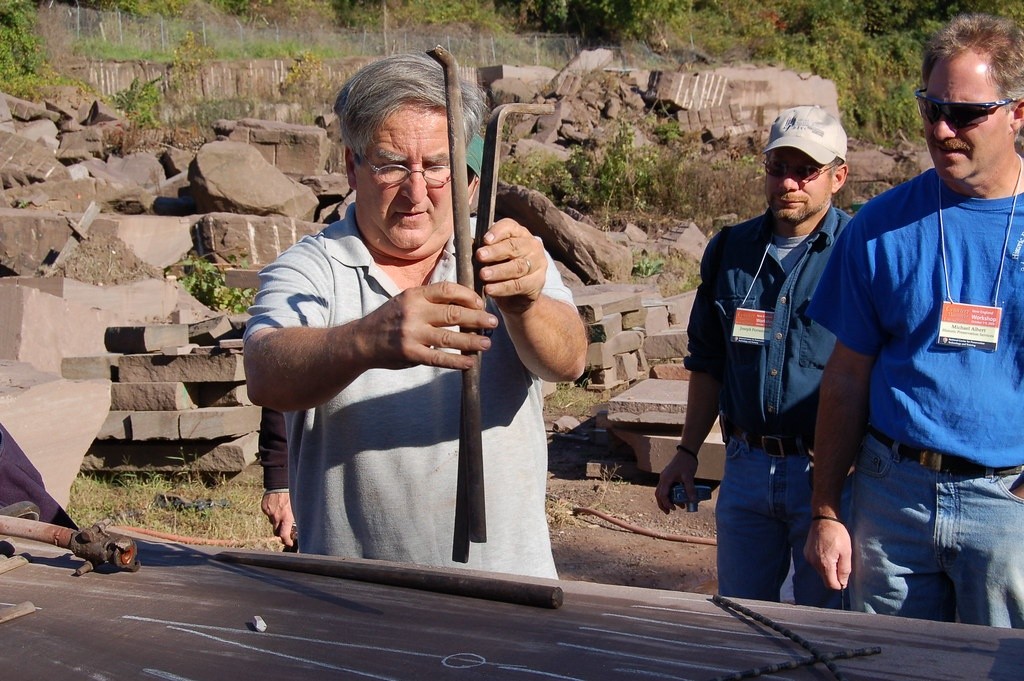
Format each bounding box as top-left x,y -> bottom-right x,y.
524,258 -> 531,275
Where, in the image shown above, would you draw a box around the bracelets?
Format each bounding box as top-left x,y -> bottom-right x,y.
812,516 -> 844,525
676,444 -> 697,459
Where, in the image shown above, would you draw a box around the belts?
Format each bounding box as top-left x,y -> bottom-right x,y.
866,423 -> 1022,479
731,422 -> 819,459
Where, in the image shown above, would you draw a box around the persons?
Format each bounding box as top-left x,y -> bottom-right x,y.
802,14 -> 1023,635
258,405 -> 300,553
656,105 -> 852,611
242,55 -> 587,583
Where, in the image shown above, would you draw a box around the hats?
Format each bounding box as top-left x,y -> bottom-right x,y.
465,136 -> 487,181
760,105 -> 849,165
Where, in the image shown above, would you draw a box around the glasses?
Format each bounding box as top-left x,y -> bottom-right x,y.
358,150 -> 452,189
762,161 -> 840,181
913,86 -> 1021,130
